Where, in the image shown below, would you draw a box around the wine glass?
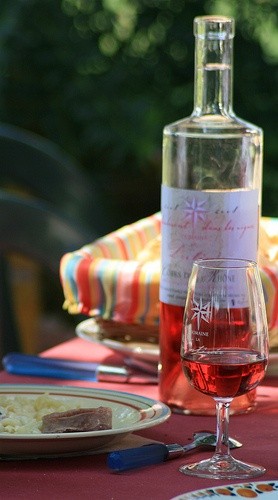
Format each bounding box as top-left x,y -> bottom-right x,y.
176,257 -> 271,482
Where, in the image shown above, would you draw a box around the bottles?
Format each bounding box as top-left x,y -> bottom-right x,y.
158,14 -> 265,415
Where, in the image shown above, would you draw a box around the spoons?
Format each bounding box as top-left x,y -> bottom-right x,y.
105,428 -> 243,474
2,352 -> 159,387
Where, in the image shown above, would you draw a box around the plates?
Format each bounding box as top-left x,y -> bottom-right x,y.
170,479 -> 278,500
75,317 -> 278,376
0,383 -> 171,456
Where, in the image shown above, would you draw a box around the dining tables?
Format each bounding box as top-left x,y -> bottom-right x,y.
0,336 -> 278,499
